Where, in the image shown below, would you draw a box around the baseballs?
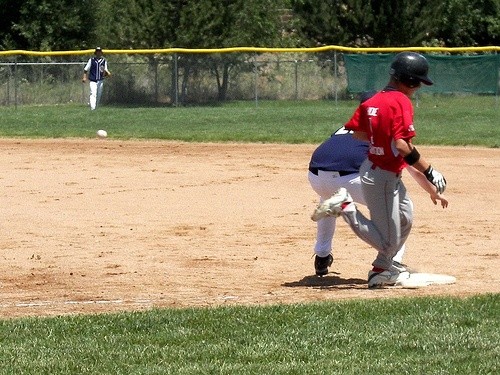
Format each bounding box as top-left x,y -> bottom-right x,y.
97,130 -> 107,137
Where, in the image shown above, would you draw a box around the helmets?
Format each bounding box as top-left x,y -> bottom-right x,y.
390,51 -> 433,88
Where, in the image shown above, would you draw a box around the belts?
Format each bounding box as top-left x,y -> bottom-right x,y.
309,167 -> 354,177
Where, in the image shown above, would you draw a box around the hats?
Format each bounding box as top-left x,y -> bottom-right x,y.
96,46 -> 102,51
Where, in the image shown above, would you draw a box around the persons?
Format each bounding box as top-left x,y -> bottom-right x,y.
308,88 -> 448,280
311,51 -> 447,290
81,46 -> 112,112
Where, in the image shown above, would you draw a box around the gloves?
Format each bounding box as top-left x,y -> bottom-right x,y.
422,165 -> 446,194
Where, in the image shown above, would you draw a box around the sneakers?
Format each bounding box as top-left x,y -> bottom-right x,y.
313,255 -> 333,275
311,188 -> 353,220
372,260 -> 410,278
367,270 -> 399,288
